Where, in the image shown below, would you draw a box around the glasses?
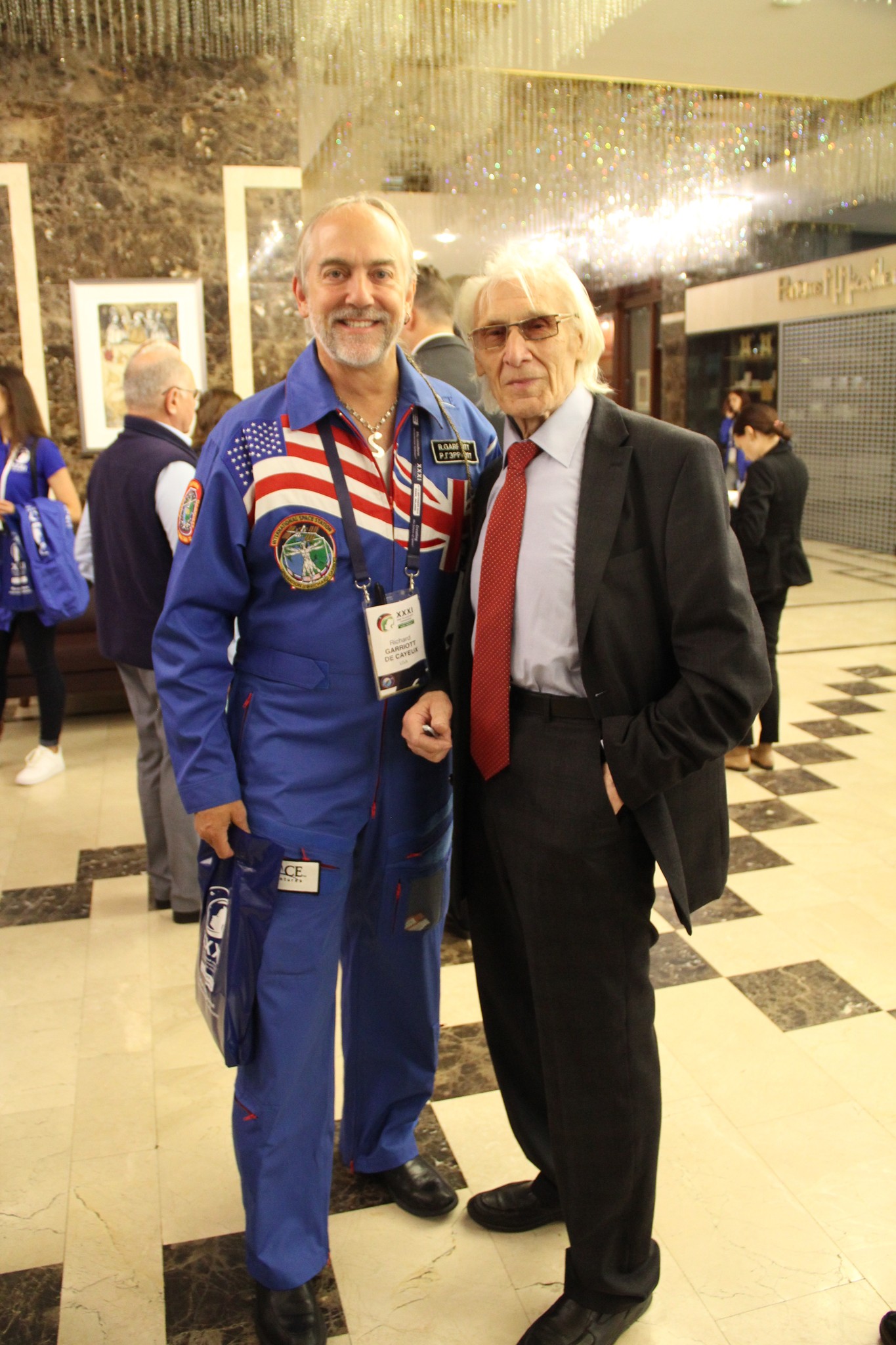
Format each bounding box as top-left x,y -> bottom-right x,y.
162,387 -> 199,399
467,313 -> 580,350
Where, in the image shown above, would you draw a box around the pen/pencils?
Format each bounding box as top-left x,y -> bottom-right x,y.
422,725 -> 439,738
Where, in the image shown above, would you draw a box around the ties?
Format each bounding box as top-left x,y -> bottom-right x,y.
469,440 -> 540,782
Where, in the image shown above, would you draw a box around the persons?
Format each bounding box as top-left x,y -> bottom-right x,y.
152,193 -> 506,1345
0,362 -> 83,787
401,233 -> 771,1345
74,341 -> 813,942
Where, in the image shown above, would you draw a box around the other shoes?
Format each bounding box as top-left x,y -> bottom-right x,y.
726,765 -> 750,771
14,744 -> 68,786
173,911 -> 202,924
150,896 -> 171,909
749,747 -> 774,770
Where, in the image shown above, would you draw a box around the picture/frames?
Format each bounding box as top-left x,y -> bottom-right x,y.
68,277 -> 207,453
0,163 -> 52,437
223,165 -> 313,401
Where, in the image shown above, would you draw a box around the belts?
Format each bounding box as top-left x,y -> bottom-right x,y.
510,684 -> 599,720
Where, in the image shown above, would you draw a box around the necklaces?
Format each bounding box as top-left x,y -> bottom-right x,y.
337,394 -> 397,458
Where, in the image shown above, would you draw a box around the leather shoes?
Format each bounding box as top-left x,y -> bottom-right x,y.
251,1278 -> 328,1345
359,1152 -> 460,1218
467,1180 -> 563,1233
515,1292 -> 653,1345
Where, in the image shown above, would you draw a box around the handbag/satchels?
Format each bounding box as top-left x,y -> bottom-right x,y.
194,820 -> 287,1067
